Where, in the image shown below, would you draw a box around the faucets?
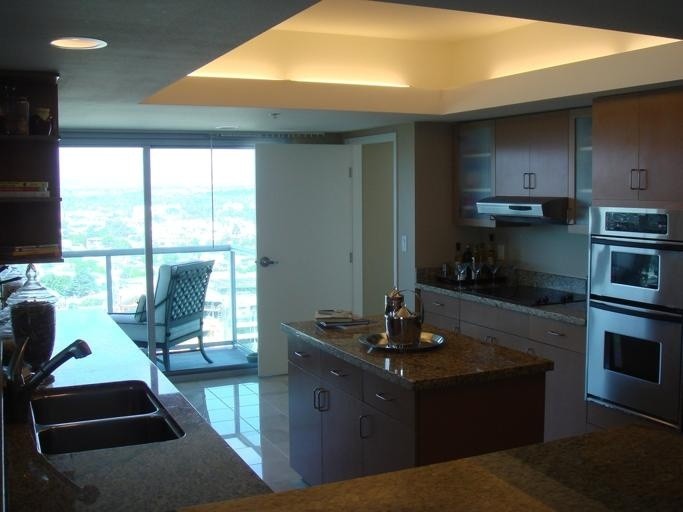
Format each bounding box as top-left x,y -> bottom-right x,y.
7,336 -> 90,423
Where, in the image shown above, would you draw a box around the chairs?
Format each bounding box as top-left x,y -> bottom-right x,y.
109,258 -> 216,372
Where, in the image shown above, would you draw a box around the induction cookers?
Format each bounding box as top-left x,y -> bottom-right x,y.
477,284 -> 586,307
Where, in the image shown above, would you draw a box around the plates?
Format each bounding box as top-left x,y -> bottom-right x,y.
357,331 -> 445,352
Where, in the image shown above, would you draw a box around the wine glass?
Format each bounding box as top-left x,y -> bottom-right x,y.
449,261 -> 499,286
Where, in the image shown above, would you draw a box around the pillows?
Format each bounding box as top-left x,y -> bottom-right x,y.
135,295 -> 153,322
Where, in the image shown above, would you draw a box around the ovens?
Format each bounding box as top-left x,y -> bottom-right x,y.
585,209 -> 683,432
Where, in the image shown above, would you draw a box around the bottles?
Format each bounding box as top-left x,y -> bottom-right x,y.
396,300 -> 412,317
452,234 -> 498,276
16,95 -> 30,136
6,264 -> 58,365
384,287 -> 405,312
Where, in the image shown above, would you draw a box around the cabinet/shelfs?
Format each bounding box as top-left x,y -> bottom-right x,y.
456,300 -> 529,352
526,313 -> 587,445
451,117 -> 497,242
591,87 -> 683,210
494,110 -> 570,206
416,287 -> 459,336
0,64 -> 62,265
287,334 -> 418,487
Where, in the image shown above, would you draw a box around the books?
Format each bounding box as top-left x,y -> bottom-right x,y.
312,307 -> 379,335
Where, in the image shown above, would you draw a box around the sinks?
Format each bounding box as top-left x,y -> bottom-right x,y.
31,380 -> 164,427
36,413 -> 185,454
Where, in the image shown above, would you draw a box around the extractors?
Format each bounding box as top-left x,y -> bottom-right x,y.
474,197 -> 566,228
